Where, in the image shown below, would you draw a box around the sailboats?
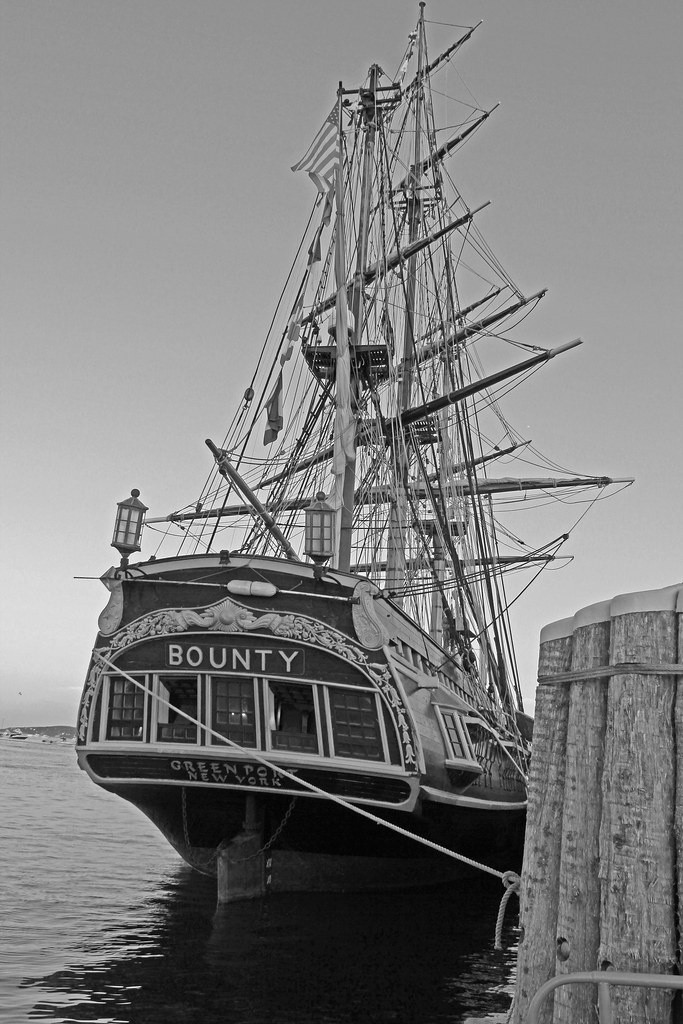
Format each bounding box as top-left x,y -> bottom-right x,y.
73,1 -> 636,877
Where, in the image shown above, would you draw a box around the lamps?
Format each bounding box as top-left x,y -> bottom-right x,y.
303,491 -> 335,579
110,487 -> 148,567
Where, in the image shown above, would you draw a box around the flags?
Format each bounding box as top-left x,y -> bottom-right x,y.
263,367 -> 285,447
306,226 -> 324,267
397,45 -> 412,94
277,287 -> 308,367
290,97 -> 341,207
